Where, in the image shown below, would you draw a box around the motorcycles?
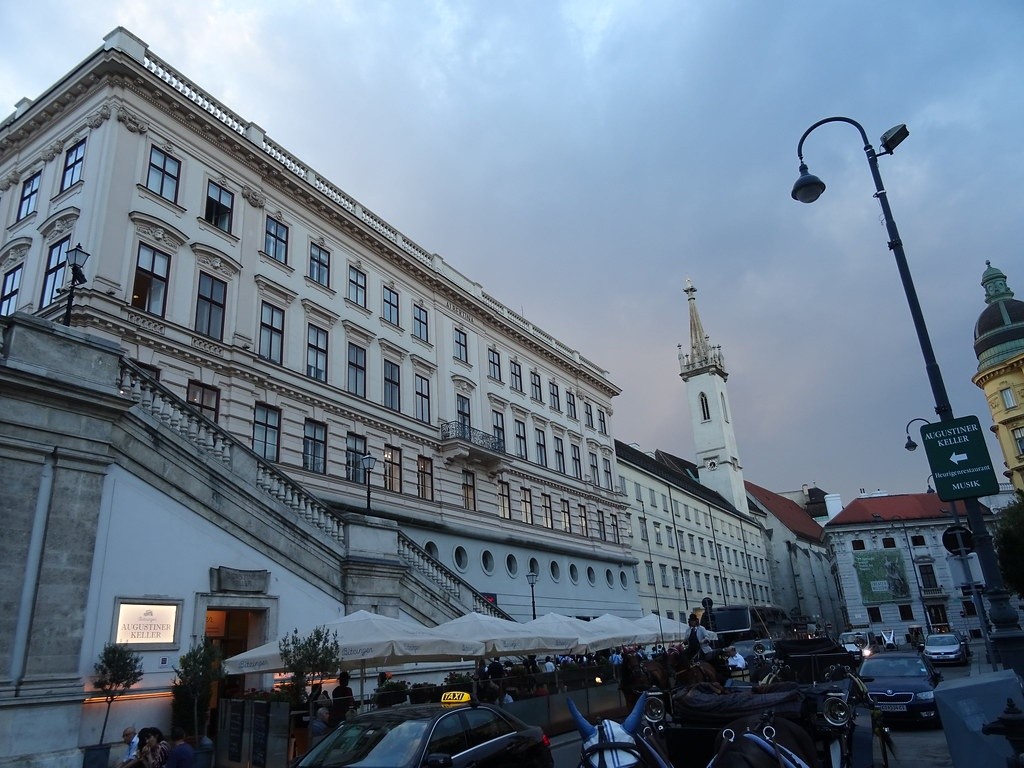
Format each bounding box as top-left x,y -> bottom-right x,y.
880,629 -> 899,651
908,624 -> 925,649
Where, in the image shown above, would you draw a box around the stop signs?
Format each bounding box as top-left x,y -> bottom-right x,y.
827,624 -> 832,629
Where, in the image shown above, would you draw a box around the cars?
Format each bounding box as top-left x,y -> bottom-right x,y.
839,631 -> 878,661
939,631 -> 970,655
857,651 -> 944,728
291,690 -> 554,768
919,634 -> 967,666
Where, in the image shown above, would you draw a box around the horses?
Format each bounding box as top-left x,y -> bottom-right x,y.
566,627 -> 817,768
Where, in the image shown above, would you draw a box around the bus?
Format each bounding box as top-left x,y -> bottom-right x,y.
700,605 -> 791,646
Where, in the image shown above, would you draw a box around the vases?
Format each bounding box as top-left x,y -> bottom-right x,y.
374,672 -> 407,708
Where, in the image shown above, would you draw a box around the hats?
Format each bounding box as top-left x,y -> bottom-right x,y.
337,672 -> 350,679
688,614 -> 697,620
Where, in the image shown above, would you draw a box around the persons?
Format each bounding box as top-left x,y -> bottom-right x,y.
728,646 -> 746,671
118,725 -> 197,767
477,644 -> 665,705
305,671 -> 389,735
679,613 -> 715,662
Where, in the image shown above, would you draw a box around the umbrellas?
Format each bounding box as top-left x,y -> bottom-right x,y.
430,611 -> 579,670
221,608 -> 486,713
524,612 -> 718,683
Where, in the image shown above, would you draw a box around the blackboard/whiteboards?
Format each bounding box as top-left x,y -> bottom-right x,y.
227,701 -> 246,763
251,703 -> 270,768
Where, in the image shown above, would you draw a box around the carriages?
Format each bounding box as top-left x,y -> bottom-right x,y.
564,643 -> 890,768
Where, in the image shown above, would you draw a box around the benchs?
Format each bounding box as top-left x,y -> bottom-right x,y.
658,678 -> 854,768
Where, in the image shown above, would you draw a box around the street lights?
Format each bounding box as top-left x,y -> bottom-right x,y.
63,243 -> 91,326
891,513 -> 933,634
789,117 -> 1024,671
904,418 -> 998,671
526,569 -> 537,620
812,615 -> 820,638
362,451 -> 377,509
927,474 -> 994,526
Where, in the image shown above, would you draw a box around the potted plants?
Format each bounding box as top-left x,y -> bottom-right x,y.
83,642 -> 146,768
408,682 -> 437,704
171,633 -> 230,768
443,671 -> 474,693
